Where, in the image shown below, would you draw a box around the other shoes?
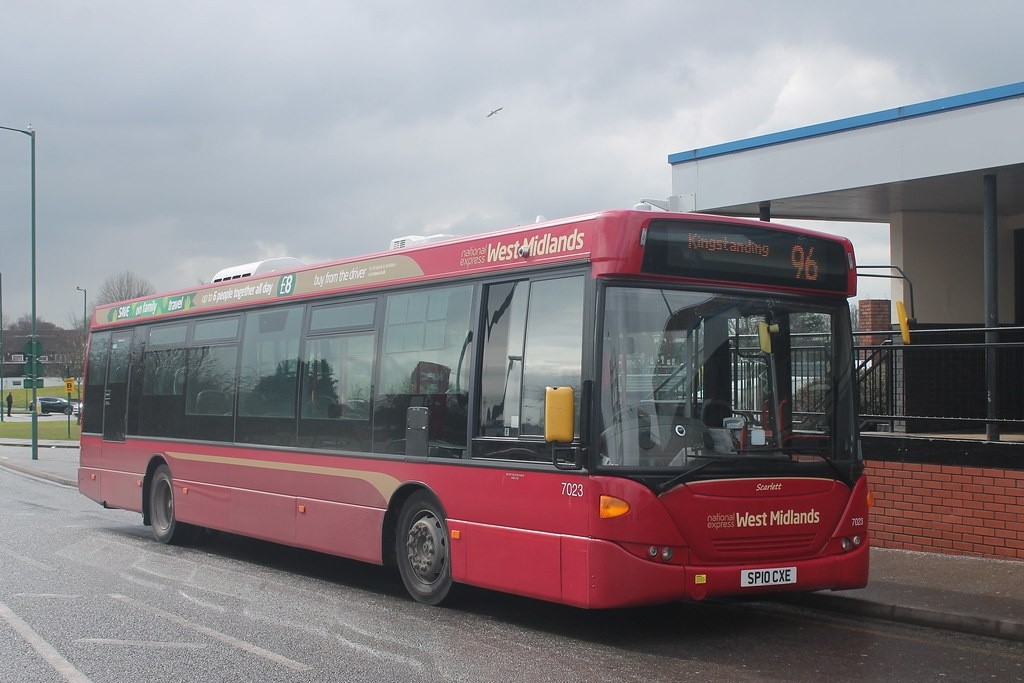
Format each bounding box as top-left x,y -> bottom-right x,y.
7,415 -> 12,417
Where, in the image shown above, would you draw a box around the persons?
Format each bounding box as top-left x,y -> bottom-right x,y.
6,392 -> 13,417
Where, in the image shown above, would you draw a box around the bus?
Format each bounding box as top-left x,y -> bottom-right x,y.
78,209 -> 871,609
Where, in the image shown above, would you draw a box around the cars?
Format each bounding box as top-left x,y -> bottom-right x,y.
29,396 -> 75,415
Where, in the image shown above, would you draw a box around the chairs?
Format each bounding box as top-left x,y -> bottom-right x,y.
141,353 -> 732,459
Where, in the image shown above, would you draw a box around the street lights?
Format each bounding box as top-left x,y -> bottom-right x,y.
76,286 -> 86,352
